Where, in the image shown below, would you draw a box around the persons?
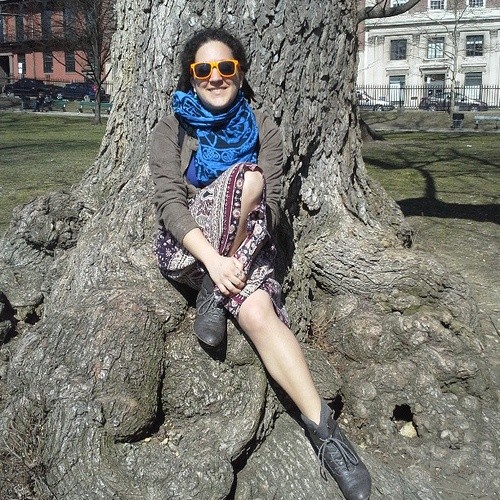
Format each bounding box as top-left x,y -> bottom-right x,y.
31,91 -> 51,112
149,27 -> 372,500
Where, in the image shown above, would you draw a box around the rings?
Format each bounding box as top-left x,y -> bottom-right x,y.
234,271 -> 243,279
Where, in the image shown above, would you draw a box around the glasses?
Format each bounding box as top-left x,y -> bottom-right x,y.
188,58 -> 240,79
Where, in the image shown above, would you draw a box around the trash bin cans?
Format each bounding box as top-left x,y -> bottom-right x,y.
452,113 -> 464,128
22,97 -> 31,109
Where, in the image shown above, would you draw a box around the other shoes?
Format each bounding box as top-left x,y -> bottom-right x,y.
193,269 -> 232,351
292,402 -> 378,496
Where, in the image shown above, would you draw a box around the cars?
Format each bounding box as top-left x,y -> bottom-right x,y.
356,90 -> 395,111
55,82 -> 107,102
418,92 -> 488,112
3,78 -> 64,100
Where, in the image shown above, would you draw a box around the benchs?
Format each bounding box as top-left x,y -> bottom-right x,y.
79,101 -> 113,114
46,100 -> 69,112
475,116 -> 499,131
